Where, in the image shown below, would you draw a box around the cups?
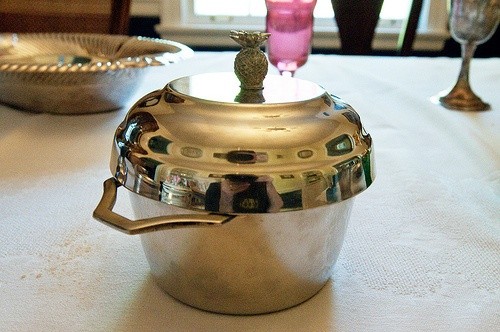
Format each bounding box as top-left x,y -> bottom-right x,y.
265,0 -> 317,77
330,0 -> 385,56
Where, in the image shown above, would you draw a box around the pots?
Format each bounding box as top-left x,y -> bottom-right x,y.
91,31 -> 377,317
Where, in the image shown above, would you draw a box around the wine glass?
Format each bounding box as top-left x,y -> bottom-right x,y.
439,0 -> 500,112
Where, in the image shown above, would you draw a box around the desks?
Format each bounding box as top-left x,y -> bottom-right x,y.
0,50 -> 499,331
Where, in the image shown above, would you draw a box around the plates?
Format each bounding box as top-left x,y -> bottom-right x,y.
0,32 -> 197,116
